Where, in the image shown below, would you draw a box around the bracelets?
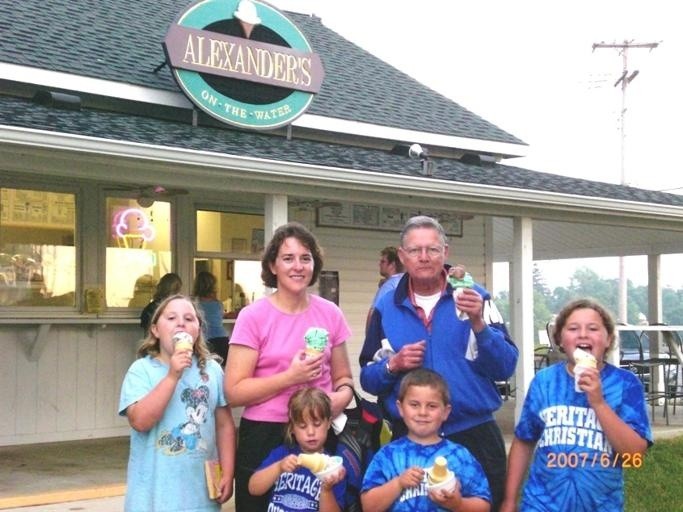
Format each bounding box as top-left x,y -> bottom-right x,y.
387,364 -> 393,374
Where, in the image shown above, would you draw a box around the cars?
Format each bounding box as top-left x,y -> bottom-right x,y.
615,323 -> 680,397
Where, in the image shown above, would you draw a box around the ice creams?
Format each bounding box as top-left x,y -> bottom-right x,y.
116,208 -> 155,249
571,347 -> 598,393
445,266 -> 475,319
172,332 -> 195,357
110,209 -> 126,248
428,456 -> 447,489
304,326 -> 330,360
233,0 -> 260,39
297,452 -> 334,474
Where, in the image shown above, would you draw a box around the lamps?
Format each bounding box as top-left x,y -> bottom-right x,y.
136,194 -> 156,209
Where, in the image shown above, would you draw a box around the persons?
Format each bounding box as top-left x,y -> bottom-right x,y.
248,388 -> 347,512
140,272 -> 182,339
223,221 -> 355,512
500,299 -> 655,512
359,369 -> 493,512
358,214 -> 520,512
192,272 -> 230,366
366,246 -> 405,335
118,294 -> 237,512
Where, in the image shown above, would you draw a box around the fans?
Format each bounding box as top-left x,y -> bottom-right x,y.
102,182 -> 191,201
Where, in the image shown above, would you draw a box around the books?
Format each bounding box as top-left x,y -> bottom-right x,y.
205,460 -> 222,500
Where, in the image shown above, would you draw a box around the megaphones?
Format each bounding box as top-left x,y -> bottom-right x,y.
409,143 -> 427,161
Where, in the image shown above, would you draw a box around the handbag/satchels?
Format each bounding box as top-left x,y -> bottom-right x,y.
333,385 -> 384,495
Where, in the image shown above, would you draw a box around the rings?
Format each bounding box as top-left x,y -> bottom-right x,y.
312,370 -> 316,377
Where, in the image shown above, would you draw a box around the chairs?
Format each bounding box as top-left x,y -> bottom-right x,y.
495,314 -> 682,428
